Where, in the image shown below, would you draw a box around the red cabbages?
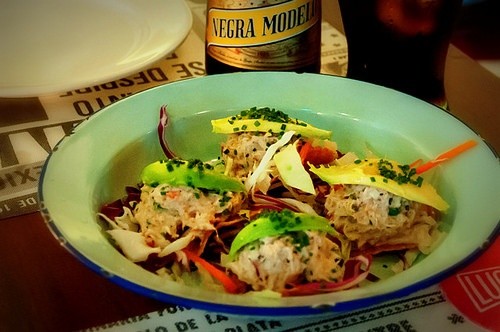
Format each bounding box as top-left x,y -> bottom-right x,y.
98,103 -> 372,295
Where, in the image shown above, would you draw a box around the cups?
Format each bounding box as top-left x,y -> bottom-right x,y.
338,0 -> 464,112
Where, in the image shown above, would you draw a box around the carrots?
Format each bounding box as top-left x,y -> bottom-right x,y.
415,140 -> 477,174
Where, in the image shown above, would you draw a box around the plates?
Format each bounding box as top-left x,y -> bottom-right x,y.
1,1 -> 194,100
36,71 -> 500,319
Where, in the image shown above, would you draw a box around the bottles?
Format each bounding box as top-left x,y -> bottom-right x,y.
205,0 -> 321,75
